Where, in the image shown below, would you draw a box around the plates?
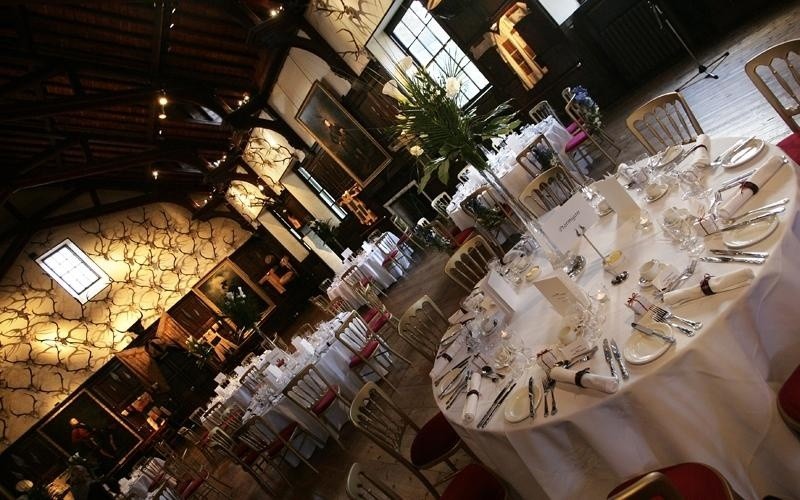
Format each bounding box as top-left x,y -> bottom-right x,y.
621,134 -> 781,364
434,318 -> 676,425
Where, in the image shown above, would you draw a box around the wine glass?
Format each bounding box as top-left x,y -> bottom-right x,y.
568,144 -> 706,260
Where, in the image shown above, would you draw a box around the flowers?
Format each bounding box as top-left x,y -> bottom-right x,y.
379,55 -> 542,225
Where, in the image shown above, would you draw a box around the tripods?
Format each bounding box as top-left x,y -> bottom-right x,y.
649,0 -> 730,92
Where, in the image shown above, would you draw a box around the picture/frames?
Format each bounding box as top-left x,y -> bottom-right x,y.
290,76 -> 393,193
35,386 -> 144,480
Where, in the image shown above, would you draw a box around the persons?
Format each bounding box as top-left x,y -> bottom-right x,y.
323,119 -> 367,161
220,280 -> 248,304
70,417 -> 118,459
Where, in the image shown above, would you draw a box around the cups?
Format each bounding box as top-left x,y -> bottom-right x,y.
451,115 -> 553,208
117,456 -> 151,495
197,320 -> 347,415
458,244 -> 610,380
